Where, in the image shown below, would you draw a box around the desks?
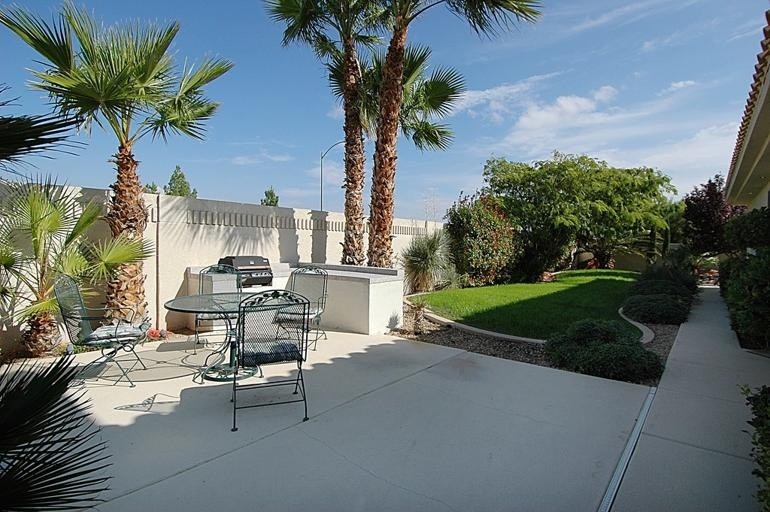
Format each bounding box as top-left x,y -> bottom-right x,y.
164,292 -> 291,384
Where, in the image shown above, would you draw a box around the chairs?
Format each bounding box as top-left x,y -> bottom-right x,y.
231,289 -> 310,432
53,274 -> 152,388
275,264 -> 328,351
193,262 -> 243,355
